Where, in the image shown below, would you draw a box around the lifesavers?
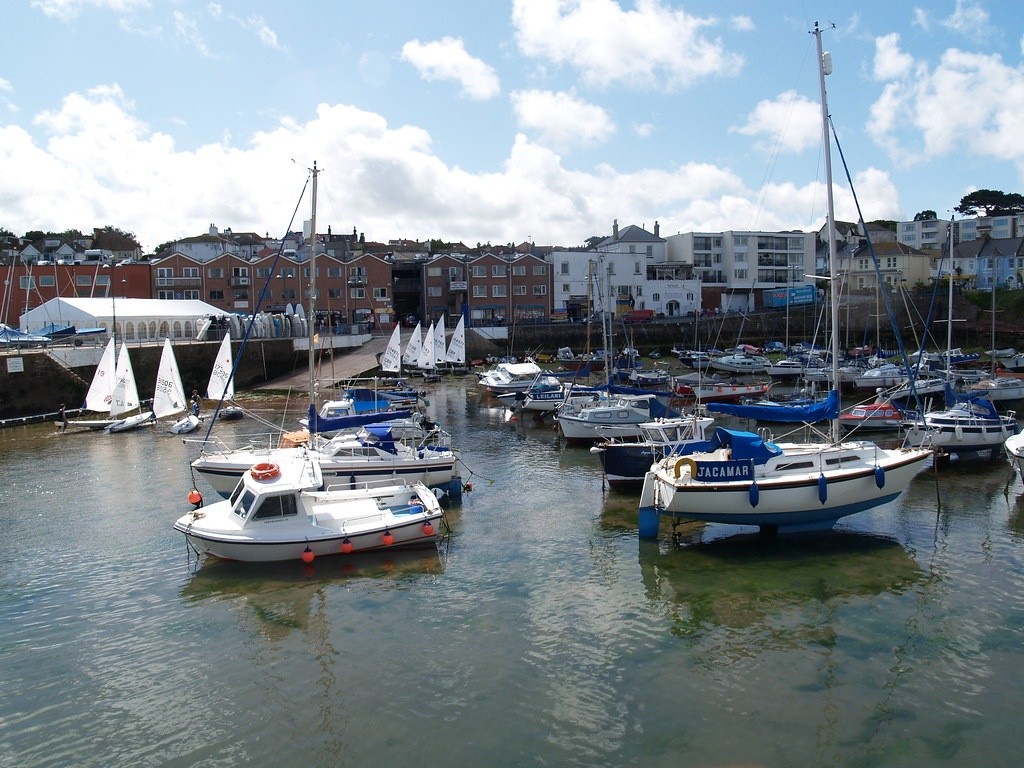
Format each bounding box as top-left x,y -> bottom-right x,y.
250,462 -> 280,481
674,457 -> 698,479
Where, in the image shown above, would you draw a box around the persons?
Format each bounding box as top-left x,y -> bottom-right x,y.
149,398 -> 157,423
56,404 -> 68,433
191,390 -> 202,417
189,400 -> 199,417
694,304 -> 736,317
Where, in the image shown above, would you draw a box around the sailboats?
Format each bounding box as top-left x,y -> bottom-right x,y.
376,310 -> 471,386
190,160 -> 464,514
203,327 -> 243,422
638,19 -> 949,543
103,339 -> 153,435
55,331 -> 159,429
151,331 -> 200,436
476,214 -> 1024,494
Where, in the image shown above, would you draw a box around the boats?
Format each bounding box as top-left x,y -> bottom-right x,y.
303,377 -> 427,430
172,441 -> 452,563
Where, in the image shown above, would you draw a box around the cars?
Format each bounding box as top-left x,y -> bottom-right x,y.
582,313 -> 603,325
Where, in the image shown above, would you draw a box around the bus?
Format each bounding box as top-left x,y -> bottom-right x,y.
931,273 -> 977,291
759,284 -> 820,311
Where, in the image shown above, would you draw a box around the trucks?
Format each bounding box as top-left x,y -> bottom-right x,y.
620,309 -> 654,324
